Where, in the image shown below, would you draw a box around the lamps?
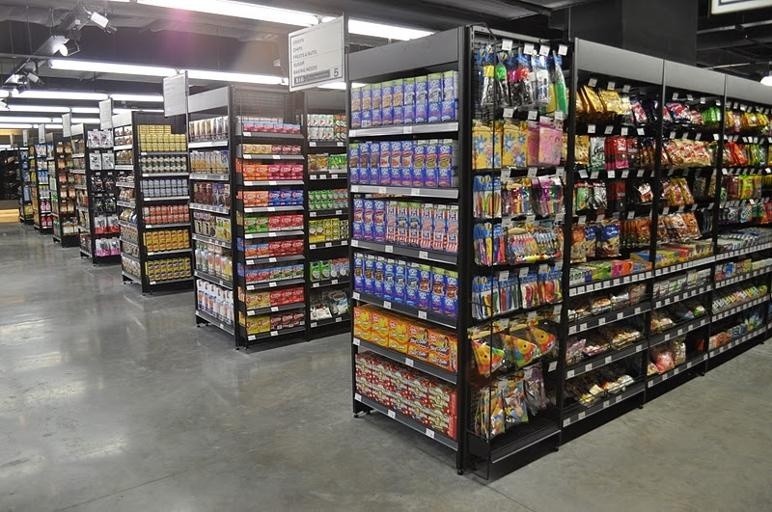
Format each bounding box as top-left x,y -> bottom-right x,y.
78,0 -> 119,39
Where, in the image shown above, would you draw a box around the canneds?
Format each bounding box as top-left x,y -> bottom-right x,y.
138,124 -> 192,281
